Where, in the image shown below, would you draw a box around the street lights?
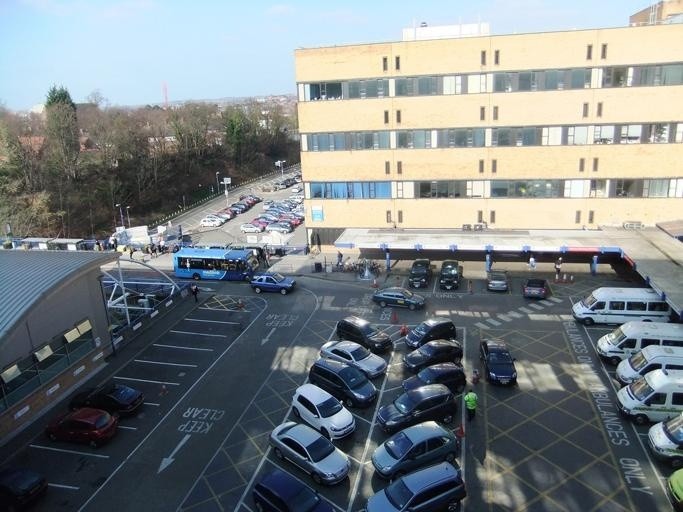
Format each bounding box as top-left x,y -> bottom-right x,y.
115,203 -> 124,228
123,205 -> 132,229
215,171 -> 221,196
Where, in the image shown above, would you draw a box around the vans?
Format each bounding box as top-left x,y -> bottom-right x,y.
615,343 -> 682,385
405,256 -> 432,289
437,259 -> 461,291
613,370 -> 682,422
595,322 -> 682,362
649,412 -> 682,460
573,287 -> 672,327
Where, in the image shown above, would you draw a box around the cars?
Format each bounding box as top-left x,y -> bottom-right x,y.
262,171 -> 302,193
287,315 -> 469,422
665,465 -> 682,507
485,268 -> 508,293
372,286 -> 425,311
480,339 -> 518,385
200,194 -> 263,227
254,421 -> 470,512
45,383 -> 148,447
519,276 -> 547,301
241,198 -> 304,234
251,273 -> 295,296
0,465 -> 47,512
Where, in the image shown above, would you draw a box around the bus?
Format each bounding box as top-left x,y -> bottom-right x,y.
173,247 -> 257,281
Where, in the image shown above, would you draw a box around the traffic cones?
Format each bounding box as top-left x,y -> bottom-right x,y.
390,312 -> 406,336
371,280 -> 378,287
554,273 -> 575,284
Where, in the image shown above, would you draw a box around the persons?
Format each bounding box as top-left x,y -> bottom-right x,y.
263,245 -> 271,268
337,258 -> 381,275
470,369 -> 481,386
554,258 -> 564,274
464,388 -> 479,423
399,325 -> 408,337
467,279 -> 475,295
94,237 -> 178,259
190,283 -> 199,302
177,257 -> 248,270
